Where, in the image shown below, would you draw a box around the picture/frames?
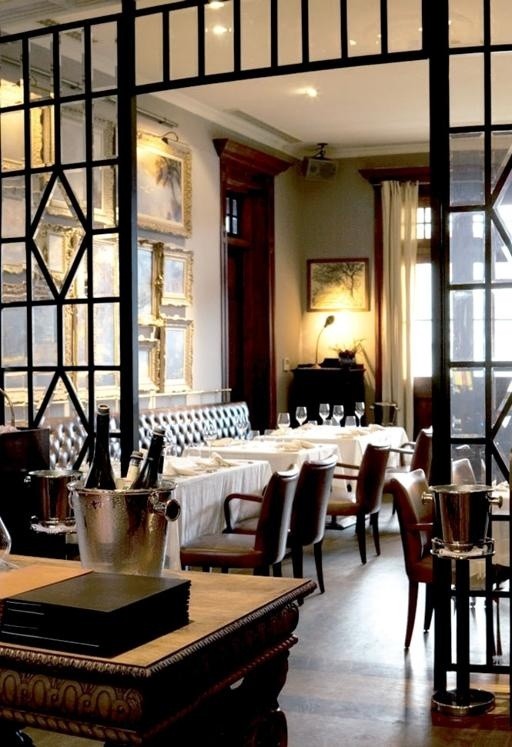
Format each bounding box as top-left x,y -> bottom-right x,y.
306,258 -> 371,312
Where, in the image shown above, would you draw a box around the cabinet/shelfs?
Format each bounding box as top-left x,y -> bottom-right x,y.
287,363 -> 368,429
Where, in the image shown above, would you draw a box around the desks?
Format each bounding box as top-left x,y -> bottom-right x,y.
1,554 -> 318,746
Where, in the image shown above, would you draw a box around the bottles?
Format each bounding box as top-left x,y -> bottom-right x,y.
132,426 -> 167,489
122,452 -> 142,488
86,405 -> 116,489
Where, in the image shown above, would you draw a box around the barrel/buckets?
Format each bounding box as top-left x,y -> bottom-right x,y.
420,485 -> 503,551
65,478 -> 182,576
369,402 -> 399,426
23,469 -> 83,528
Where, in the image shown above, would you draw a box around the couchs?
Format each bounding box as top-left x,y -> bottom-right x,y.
29,402 -> 248,508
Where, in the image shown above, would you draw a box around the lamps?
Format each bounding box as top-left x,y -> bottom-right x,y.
311,316 -> 335,370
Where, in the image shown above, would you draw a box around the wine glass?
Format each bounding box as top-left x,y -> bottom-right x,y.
168,400 -> 365,483
